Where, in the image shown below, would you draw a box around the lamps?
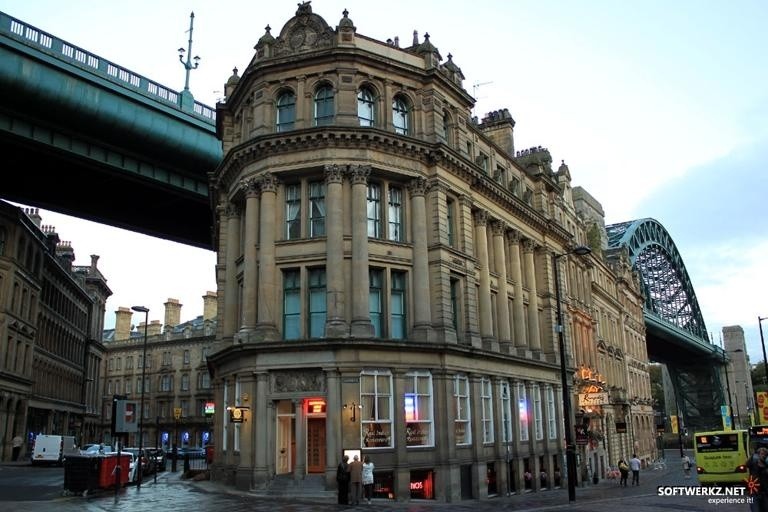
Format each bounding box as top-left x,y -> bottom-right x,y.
343,404 -> 363,422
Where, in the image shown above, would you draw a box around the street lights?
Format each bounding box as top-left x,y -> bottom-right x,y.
549,244 -> 593,503
131,305 -> 150,488
722,349 -> 744,431
177,10 -> 201,89
673,371 -> 689,458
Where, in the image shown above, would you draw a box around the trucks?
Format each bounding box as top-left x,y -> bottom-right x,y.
30,432 -> 82,467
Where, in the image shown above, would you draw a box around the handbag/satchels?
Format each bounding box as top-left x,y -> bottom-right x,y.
620,463 -> 629,471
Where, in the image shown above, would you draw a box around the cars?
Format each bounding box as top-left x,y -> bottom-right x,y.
165,445 -> 205,460
107,450 -> 138,483
80,441 -> 168,472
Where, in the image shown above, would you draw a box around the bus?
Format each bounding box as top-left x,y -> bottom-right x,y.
749,424 -> 768,451
692,428 -> 750,490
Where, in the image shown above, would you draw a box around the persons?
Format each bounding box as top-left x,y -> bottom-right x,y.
616,455 -> 629,487
345,455 -> 363,507
629,454 -> 641,486
743,447 -> 767,512
361,455 -> 376,506
5,432 -> 24,461
334,455 -> 349,507
679,452 -> 692,480
